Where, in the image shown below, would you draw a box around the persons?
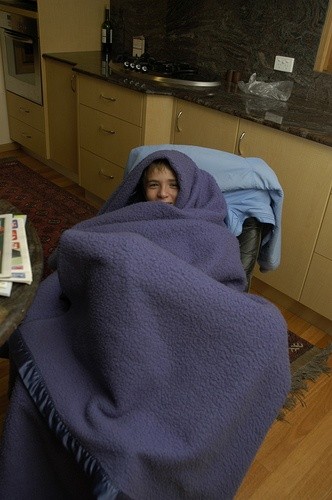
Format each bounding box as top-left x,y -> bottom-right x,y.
140,157 -> 179,206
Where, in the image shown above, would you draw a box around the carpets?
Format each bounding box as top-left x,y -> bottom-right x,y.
0,151 -> 331,420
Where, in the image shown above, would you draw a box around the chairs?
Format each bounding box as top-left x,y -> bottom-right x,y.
0,139 -> 284,403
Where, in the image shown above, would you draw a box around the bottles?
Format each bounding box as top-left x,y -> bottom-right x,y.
101,9 -> 113,53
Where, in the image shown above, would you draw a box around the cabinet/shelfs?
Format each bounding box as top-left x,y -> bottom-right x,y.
75,72 -> 173,204
0,0 -> 105,161
170,96 -> 239,153
234,118 -> 332,302
296,192 -> 332,320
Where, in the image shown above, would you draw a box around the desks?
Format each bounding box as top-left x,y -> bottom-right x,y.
0,193 -> 45,397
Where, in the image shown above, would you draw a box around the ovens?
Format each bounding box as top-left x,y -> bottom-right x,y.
0,10 -> 42,106
